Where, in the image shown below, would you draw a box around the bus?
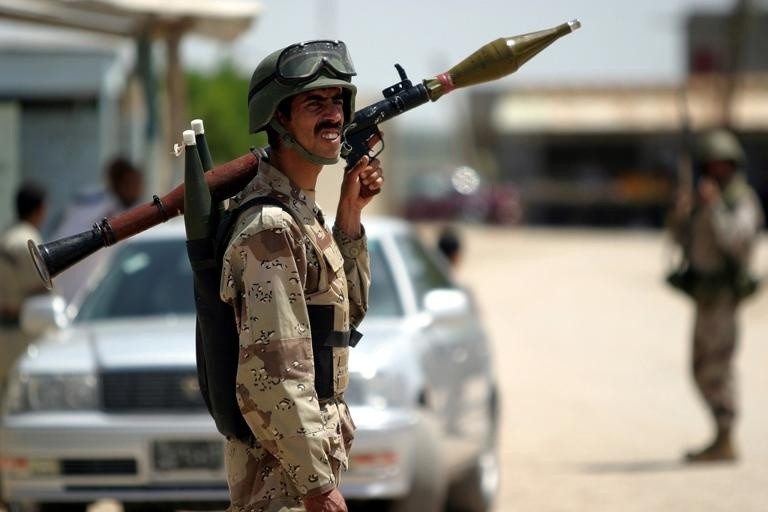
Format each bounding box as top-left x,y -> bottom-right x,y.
491,90 -> 767,226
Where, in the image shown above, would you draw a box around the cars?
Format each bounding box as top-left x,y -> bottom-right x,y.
404,169 -> 522,226
1,215 -> 502,510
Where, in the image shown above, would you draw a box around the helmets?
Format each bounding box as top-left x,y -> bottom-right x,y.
691,129 -> 750,170
245,47 -> 357,134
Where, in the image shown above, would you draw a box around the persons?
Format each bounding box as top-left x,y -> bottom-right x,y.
665,128 -> 757,463
215,40 -> 384,511
0,152 -> 144,333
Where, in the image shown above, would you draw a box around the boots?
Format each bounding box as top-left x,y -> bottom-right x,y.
685,431 -> 737,463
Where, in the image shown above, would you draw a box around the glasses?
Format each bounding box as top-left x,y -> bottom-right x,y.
247,39 -> 359,106
